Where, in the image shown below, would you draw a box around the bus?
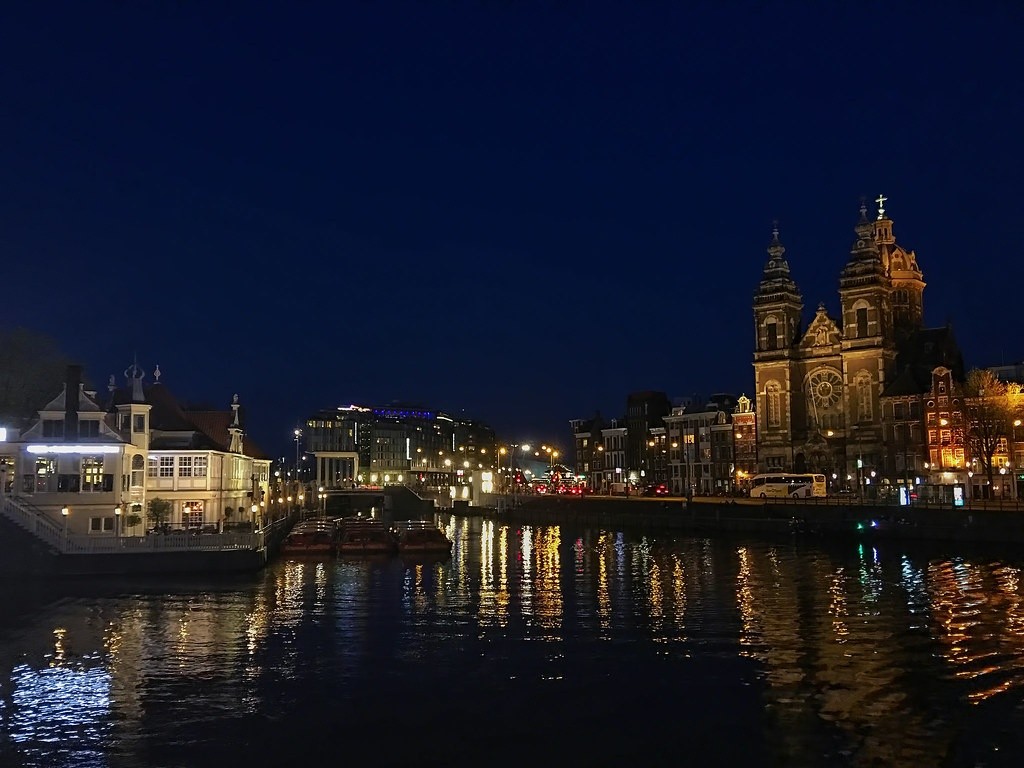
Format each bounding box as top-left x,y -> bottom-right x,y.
750,472 -> 827,499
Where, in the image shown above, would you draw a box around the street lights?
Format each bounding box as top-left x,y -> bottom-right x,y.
294,429 -> 301,478
542,445 -> 558,465
497,446 -> 506,469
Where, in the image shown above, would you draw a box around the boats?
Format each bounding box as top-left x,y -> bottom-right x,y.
283,515 -> 454,551
